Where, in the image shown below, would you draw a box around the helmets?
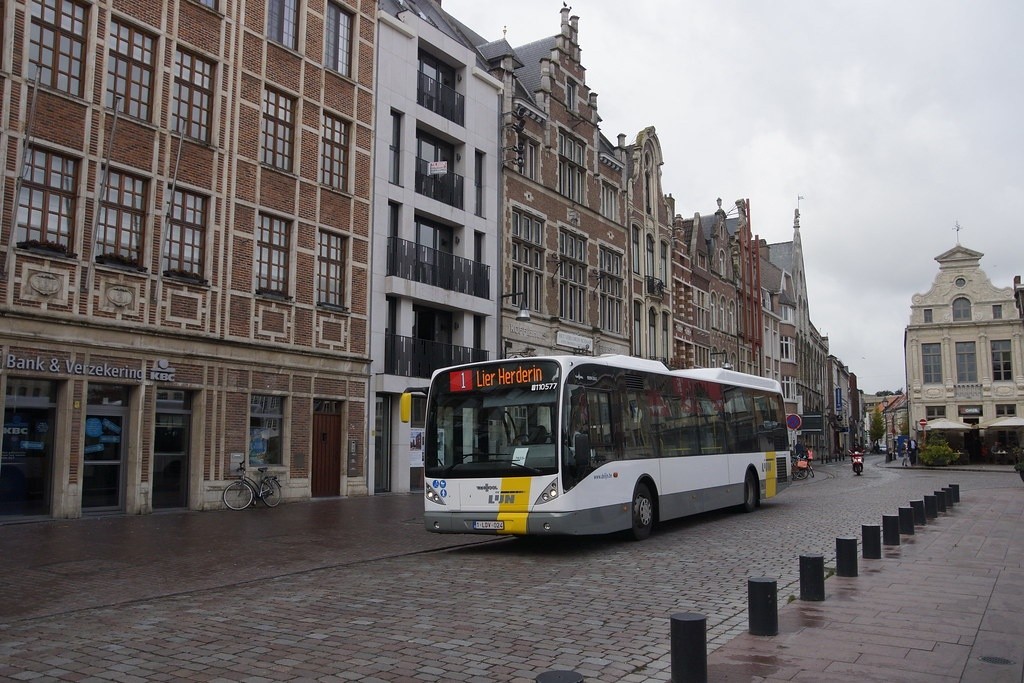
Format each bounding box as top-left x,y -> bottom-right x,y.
854,442 -> 860,449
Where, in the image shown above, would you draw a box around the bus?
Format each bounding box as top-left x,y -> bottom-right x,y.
400,353 -> 793,543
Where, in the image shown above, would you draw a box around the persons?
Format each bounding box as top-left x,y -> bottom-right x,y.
872,442 -> 881,454
788,440 -> 814,464
901,438 -> 911,467
908,436 -> 918,466
851,442 -> 864,454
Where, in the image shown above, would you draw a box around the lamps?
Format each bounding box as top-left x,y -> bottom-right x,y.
501,292 -> 531,321
710,352 -> 731,368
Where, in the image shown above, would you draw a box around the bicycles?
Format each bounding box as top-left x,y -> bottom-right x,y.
791,454 -> 815,480
223,460 -> 282,510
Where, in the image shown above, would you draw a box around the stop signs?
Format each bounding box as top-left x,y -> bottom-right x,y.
919,418 -> 927,427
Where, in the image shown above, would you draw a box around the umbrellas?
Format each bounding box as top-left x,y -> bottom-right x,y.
972,417 -> 1024,448
917,417 -> 971,443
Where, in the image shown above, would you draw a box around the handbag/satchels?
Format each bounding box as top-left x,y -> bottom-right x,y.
901,449 -> 907,453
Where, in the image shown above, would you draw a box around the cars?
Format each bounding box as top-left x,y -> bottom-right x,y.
880,445 -> 886,453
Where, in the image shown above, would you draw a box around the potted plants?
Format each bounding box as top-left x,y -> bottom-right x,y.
918,430 -> 953,465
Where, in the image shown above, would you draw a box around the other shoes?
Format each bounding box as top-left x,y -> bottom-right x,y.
905,465 -> 907,467
902,463 -> 904,465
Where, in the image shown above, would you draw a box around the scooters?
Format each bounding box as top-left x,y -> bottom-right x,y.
847,449 -> 866,475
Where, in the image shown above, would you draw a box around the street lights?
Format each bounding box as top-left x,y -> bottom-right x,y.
881,396 -> 890,463
